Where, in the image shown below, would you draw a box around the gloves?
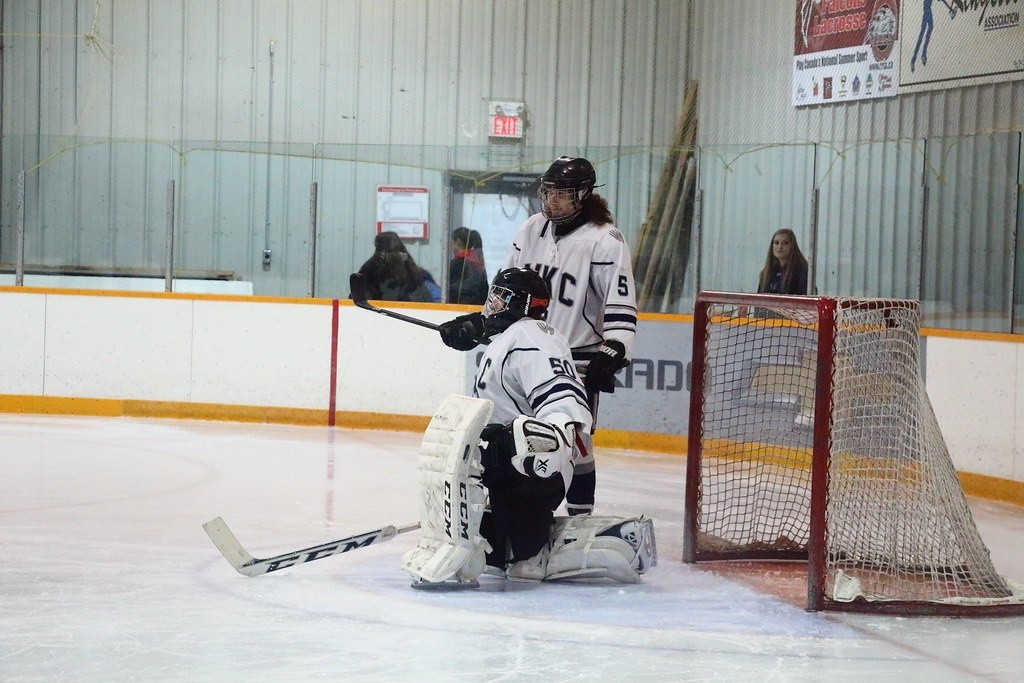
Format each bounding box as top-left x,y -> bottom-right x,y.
439,312 -> 487,351
585,340 -> 630,395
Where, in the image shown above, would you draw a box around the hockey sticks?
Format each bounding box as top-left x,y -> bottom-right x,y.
200,514 -> 423,578
348,272 -> 618,386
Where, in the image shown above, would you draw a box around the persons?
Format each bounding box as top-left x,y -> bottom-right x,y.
349,231 -> 441,303
440,156 -> 638,517
754,228 -> 818,318
399,267 -> 657,587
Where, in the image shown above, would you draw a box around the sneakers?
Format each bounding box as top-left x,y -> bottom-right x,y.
620,518 -> 658,575
411,576 -> 481,588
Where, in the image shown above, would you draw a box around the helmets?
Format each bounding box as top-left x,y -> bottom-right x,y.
480,267 -> 550,330
538,156 -> 596,225
